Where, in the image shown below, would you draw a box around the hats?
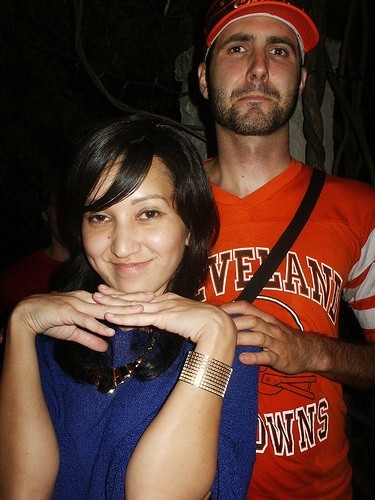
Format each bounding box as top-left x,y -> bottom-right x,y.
202,0 -> 320,64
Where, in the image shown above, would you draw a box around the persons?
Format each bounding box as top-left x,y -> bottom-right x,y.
178,0 -> 375,500
0,112 -> 242,499
0,177 -> 65,338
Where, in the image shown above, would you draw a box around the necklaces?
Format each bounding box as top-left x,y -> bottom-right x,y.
87,326 -> 159,394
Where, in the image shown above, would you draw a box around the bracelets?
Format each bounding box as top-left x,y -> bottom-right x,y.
179,350 -> 235,398
